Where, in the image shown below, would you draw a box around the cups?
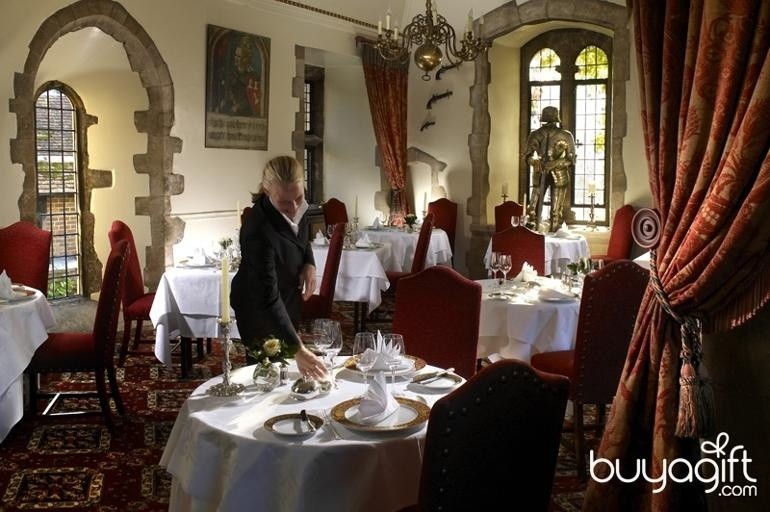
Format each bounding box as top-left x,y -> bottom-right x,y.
510,215 -> 528,228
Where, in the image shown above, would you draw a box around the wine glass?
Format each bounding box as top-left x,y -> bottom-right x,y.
379,213 -> 387,230
540,217 -> 550,236
311,318 -> 344,389
327,224 -> 351,249
211,237 -> 241,272
351,331 -> 405,399
489,252 -> 603,303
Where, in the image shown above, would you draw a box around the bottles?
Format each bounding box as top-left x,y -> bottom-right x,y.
279,363 -> 289,385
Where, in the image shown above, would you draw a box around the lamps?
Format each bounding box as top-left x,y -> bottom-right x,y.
375,0 -> 486,81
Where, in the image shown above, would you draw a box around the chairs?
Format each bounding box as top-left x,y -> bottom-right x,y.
24,237 -> 133,446
0,218 -> 53,304
108,198 -> 659,512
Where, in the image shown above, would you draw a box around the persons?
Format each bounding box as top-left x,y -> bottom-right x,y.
523,106 -> 576,233
215,37 -> 257,118
229,157 -> 328,385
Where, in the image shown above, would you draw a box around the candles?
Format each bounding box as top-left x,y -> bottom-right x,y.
588,182 -> 596,194
501,184 -> 508,196
423,192 -> 427,211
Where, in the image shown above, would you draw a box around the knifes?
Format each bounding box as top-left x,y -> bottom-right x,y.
301,409 -> 316,432
407,366 -> 457,389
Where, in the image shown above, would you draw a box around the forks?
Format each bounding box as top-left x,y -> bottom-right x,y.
321,409 -> 344,441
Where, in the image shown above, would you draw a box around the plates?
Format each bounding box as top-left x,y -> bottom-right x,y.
0,285 -> 35,305
365,226 -> 426,232
330,397 -> 431,433
343,354 -> 425,376
177,254 -> 220,268
357,241 -> 383,249
264,415 -> 324,436
313,238 -> 331,246
414,373 -> 461,390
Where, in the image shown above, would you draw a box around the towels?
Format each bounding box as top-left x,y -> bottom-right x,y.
0,265 -> 16,300
